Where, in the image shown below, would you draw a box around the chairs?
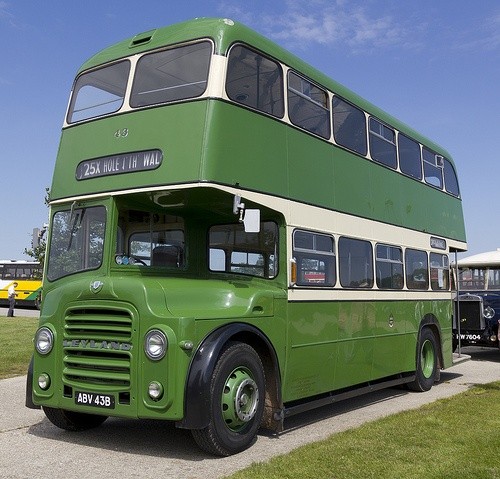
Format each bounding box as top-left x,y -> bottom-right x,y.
150,246 -> 180,268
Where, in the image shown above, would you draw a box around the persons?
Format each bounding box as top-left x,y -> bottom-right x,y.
7,282 -> 18,317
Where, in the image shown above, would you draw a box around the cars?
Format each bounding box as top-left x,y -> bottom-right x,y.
452,249 -> 500,355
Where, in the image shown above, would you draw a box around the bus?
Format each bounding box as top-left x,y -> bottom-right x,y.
25,18 -> 467,456
0,259 -> 43,310
